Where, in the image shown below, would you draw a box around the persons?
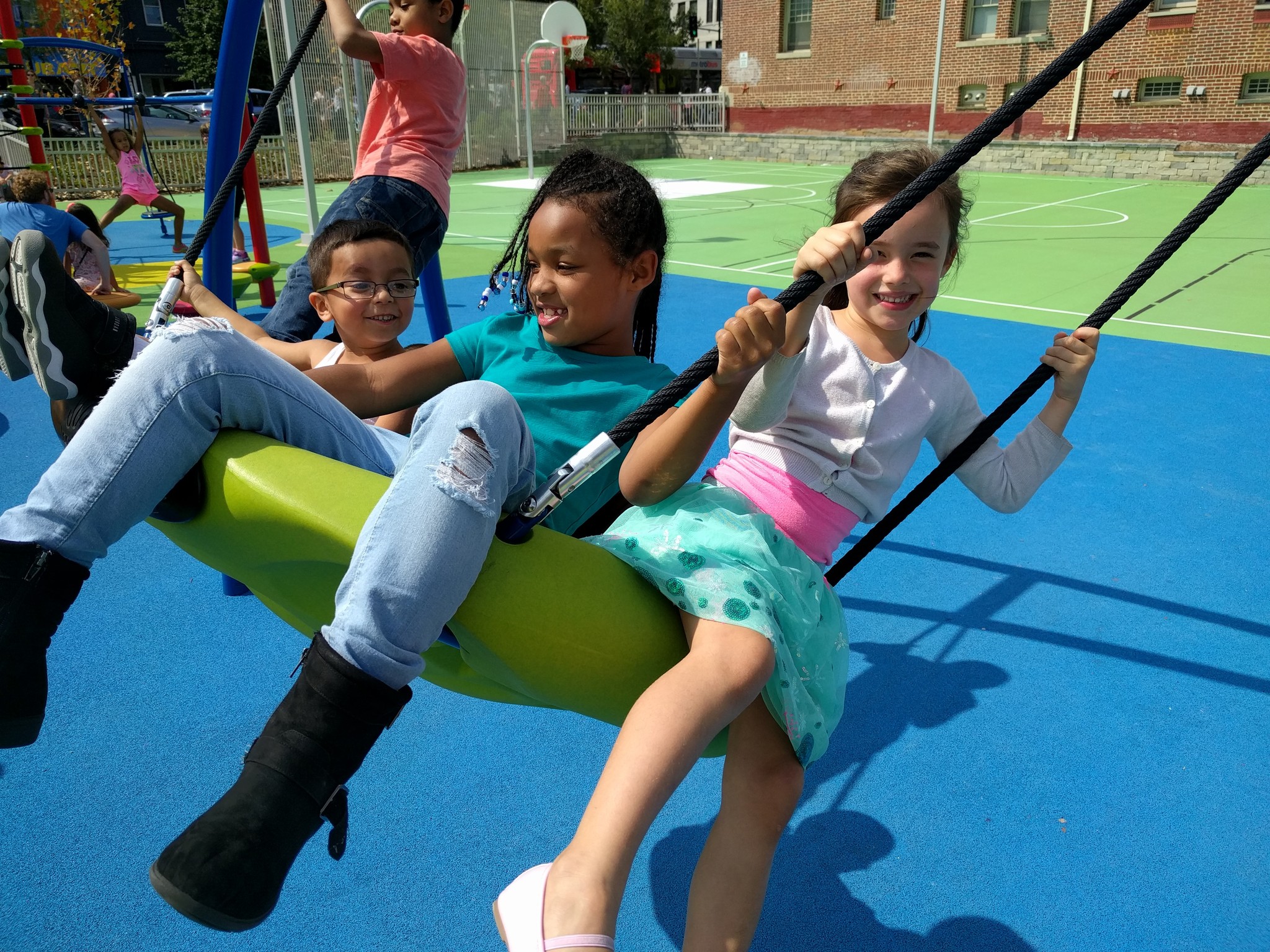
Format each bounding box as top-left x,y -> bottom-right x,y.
201,121 -> 249,266
491,150 -> 1099,952
0,154 -> 129,295
0,220 -> 424,436
314,75 -> 347,140
621,80 -> 632,94
259,0 -> 469,347
0,151 -> 787,933
71,68 -> 96,138
27,69 -> 50,127
87,102 -> 191,252
535,75 -> 552,134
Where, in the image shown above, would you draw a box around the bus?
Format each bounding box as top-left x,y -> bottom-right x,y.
522,48 -> 661,110
655,47 -> 722,95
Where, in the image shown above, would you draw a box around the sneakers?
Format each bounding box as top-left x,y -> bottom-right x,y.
0,236 -> 34,381
232,251 -> 250,264
10,229 -> 136,447
172,243 -> 188,253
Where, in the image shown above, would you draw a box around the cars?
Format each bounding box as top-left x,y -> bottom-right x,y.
0,87 -> 349,146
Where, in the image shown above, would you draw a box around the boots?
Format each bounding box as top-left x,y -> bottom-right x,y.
0,540 -> 91,750
149,633 -> 413,932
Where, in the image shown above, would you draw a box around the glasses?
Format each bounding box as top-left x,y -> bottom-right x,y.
316,279 -> 419,299
47,188 -> 53,193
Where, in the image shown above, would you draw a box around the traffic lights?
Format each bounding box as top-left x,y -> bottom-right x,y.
689,16 -> 697,38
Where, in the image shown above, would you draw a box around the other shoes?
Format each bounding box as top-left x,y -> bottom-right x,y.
492,861 -> 614,952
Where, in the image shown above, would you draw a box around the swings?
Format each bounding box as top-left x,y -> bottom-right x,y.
145,0 -> 1270,755
